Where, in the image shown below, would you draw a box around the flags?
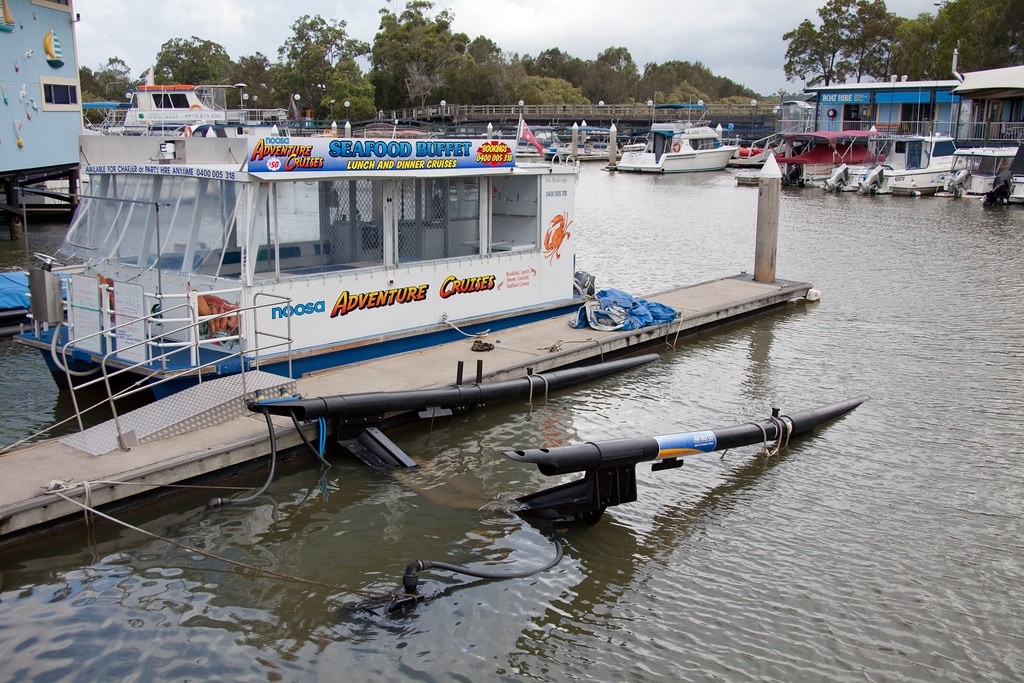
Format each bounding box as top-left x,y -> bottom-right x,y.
520,120 -> 543,157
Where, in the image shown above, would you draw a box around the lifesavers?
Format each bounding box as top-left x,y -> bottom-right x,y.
673,143 -> 681,153
185,126 -> 192,137
190,104 -> 201,110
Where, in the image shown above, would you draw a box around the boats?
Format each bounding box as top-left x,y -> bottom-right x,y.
7,82 -> 1024,400
